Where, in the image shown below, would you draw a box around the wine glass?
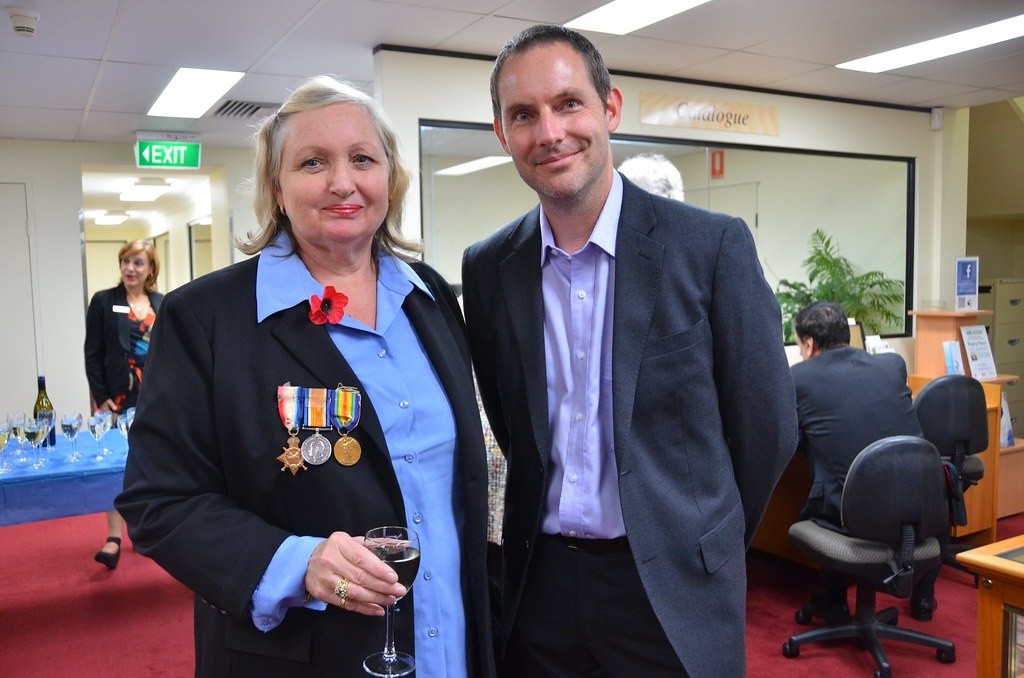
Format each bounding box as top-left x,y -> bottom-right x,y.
361,526 -> 420,678
87,409 -> 113,464
0,412 -> 58,475
60,410 -> 83,463
117,406 -> 135,464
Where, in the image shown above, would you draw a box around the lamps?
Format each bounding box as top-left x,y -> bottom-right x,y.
120,178 -> 171,201
95,209 -> 130,226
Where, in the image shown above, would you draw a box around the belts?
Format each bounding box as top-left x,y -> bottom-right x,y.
551,535 -> 629,552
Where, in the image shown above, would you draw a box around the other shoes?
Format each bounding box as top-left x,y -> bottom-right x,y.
911,593 -> 937,619
813,591 -> 850,623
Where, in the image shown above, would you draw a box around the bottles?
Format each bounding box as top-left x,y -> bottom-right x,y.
32,375 -> 58,449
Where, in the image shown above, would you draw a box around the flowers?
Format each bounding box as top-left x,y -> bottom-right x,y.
307,284 -> 349,325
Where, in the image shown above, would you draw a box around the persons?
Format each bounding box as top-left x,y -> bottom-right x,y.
618,152 -> 685,202
114,73 -> 496,678
791,303 -> 938,627
83,240 -> 164,571
461,24 -> 799,678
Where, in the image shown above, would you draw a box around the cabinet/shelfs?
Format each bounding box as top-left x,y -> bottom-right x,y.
908,276 -> 1024,520
747,375 -> 1002,572
954,534 -> 1024,678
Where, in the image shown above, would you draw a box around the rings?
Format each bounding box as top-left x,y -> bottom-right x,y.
334,578 -> 350,598
340,598 -> 346,609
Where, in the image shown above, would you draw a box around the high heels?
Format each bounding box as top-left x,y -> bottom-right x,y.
95,537 -> 120,569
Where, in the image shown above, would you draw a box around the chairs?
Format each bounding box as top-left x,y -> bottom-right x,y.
777,435 -> 952,678
910,375 -> 990,588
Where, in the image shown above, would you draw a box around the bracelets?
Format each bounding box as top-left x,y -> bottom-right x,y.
304,593 -> 312,602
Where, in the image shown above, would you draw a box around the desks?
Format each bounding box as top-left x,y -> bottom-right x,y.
0,428 -> 132,526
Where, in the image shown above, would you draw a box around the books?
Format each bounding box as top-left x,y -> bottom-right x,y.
864,335 -> 895,355
